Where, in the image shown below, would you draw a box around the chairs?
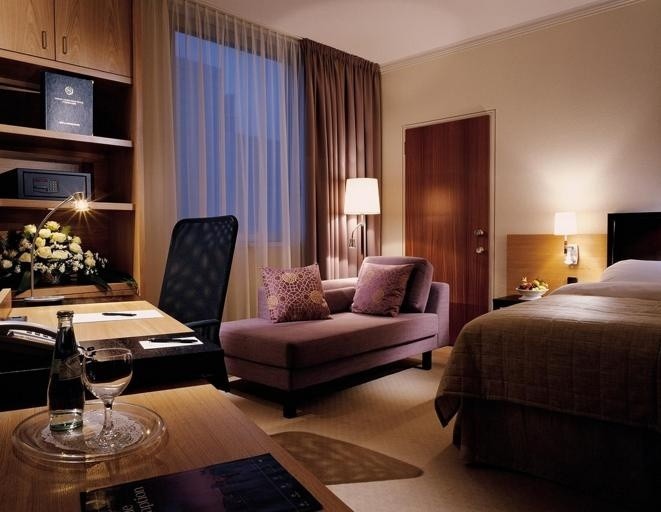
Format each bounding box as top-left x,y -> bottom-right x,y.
146,215 -> 238,391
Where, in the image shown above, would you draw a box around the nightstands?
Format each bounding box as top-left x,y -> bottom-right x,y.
493,295 -> 526,310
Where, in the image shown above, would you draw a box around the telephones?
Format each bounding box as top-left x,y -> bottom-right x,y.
0,316 -> 94,367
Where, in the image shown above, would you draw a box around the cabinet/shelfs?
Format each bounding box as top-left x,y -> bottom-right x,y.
1,55 -> 143,298
0,1 -> 133,85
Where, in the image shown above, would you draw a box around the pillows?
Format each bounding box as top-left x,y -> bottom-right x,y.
258,263 -> 331,324
358,256 -> 434,311
350,263 -> 413,317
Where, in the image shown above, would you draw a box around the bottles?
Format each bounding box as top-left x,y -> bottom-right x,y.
44,308 -> 84,435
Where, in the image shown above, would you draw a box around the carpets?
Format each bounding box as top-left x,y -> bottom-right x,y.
267,431 -> 425,484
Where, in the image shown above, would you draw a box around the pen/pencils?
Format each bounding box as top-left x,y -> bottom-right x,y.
102,312 -> 135,316
148,338 -> 198,343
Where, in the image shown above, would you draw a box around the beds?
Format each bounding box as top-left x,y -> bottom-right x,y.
434,211 -> 660,494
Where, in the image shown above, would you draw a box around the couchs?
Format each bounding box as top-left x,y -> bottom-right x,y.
220,279 -> 450,418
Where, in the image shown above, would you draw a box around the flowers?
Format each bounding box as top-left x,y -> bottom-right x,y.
1,220 -> 108,293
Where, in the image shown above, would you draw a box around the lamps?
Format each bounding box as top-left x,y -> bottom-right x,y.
344,178 -> 380,277
26,192 -> 88,302
554,211 -> 579,266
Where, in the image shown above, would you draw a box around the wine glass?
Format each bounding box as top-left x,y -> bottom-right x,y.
80,348 -> 135,447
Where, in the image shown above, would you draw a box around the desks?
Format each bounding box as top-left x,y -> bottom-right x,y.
2,299 -> 195,344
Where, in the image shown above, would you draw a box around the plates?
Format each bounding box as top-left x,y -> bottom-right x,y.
10,400 -> 167,470
518,297 -> 541,300
515,287 -> 549,298
